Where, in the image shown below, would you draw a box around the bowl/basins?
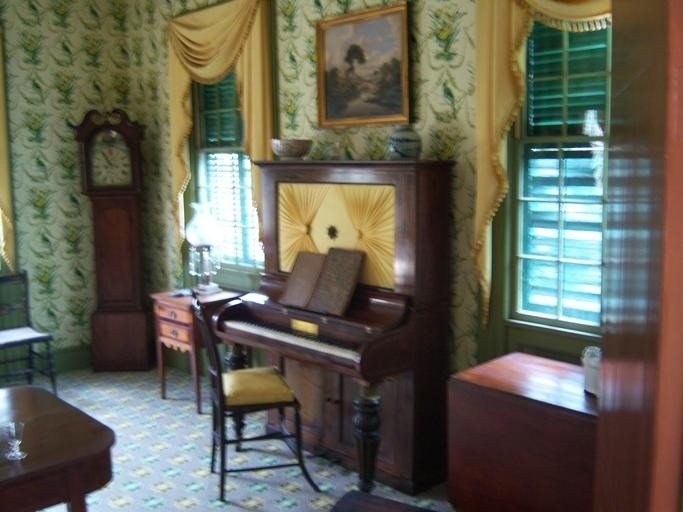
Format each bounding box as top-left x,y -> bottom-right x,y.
272,138 -> 313,161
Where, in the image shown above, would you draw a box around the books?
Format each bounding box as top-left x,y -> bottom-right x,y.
278,248 -> 365,317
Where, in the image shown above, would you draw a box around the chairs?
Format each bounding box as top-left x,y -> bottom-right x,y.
0,271 -> 60,395
190,288 -> 323,501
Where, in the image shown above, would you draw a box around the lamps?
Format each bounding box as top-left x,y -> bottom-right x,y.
185,199 -> 223,296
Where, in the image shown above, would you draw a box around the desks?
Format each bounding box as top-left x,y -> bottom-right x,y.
1,386 -> 117,512
147,285 -> 249,414
445,351 -> 653,512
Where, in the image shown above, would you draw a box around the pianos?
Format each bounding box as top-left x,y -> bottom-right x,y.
210,273 -> 420,494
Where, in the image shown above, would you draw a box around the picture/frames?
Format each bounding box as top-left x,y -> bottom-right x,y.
313,2 -> 412,130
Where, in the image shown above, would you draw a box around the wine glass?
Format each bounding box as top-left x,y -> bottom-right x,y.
0,421 -> 27,460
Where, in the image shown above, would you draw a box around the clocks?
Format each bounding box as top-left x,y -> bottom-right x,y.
69,111 -> 148,370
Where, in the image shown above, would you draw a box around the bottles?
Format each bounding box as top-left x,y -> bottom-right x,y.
389,122 -> 420,159
